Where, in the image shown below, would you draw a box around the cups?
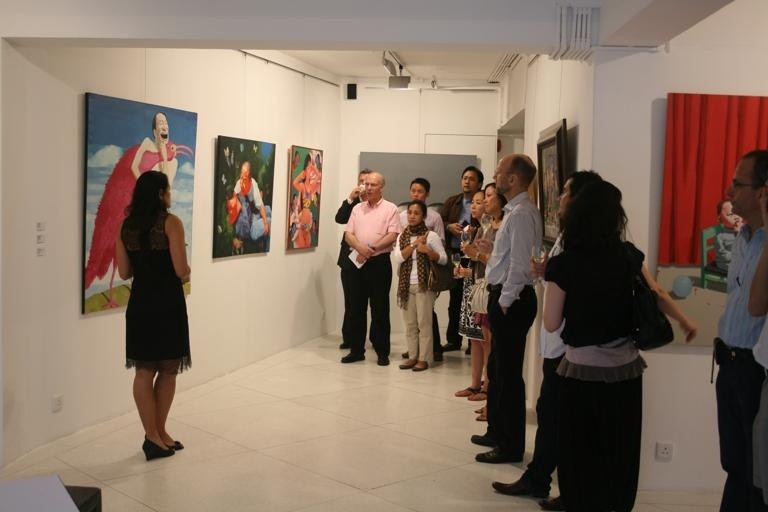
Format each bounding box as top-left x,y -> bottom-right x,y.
356,183 -> 364,197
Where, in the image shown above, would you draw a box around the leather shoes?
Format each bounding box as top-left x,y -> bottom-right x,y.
341,354 -> 365,363
475,448 -> 524,463
413,362 -> 428,370
493,478 -> 549,497
476,406 -> 487,420
377,356 -> 389,365
399,359 -> 418,369
471,433 -> 493,446
538,497 -> 561,509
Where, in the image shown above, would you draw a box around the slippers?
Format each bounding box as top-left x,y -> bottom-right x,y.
456,387 -> 487,400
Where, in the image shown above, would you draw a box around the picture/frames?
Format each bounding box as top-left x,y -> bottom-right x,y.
536,119 -> 577,247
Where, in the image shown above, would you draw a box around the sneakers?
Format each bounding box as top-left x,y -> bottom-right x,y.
441,342 -> 461,351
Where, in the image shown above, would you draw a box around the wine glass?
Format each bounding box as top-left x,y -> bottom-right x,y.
459,229 -> 472,259
531,244 -> 545,285
451,253 -> 466,280
478,213 -> 494,243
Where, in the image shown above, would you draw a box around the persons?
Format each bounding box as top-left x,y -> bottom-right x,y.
131,110 -> 178,188
233,160 -> 271,251
539,179 -> 697,512
289,151 -> 322,249
441,154 -> 542,464
493,170 -> 602,498
115,172 -> 191,461
335,169 -> 449,371
713,151 -> 768,511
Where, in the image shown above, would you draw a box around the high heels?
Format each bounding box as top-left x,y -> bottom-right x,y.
142,436 -> 185,461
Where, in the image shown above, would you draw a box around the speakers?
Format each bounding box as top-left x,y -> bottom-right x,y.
347,84 -> 356,99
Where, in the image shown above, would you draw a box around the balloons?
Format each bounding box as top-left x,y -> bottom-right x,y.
673,275 -> 692,298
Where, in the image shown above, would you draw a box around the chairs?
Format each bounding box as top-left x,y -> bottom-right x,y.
700,223 -> 729,290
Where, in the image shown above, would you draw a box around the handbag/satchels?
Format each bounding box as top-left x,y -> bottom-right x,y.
471,282 -> 489,315
628,277 -> 674,350
427,260 -> 453,291
